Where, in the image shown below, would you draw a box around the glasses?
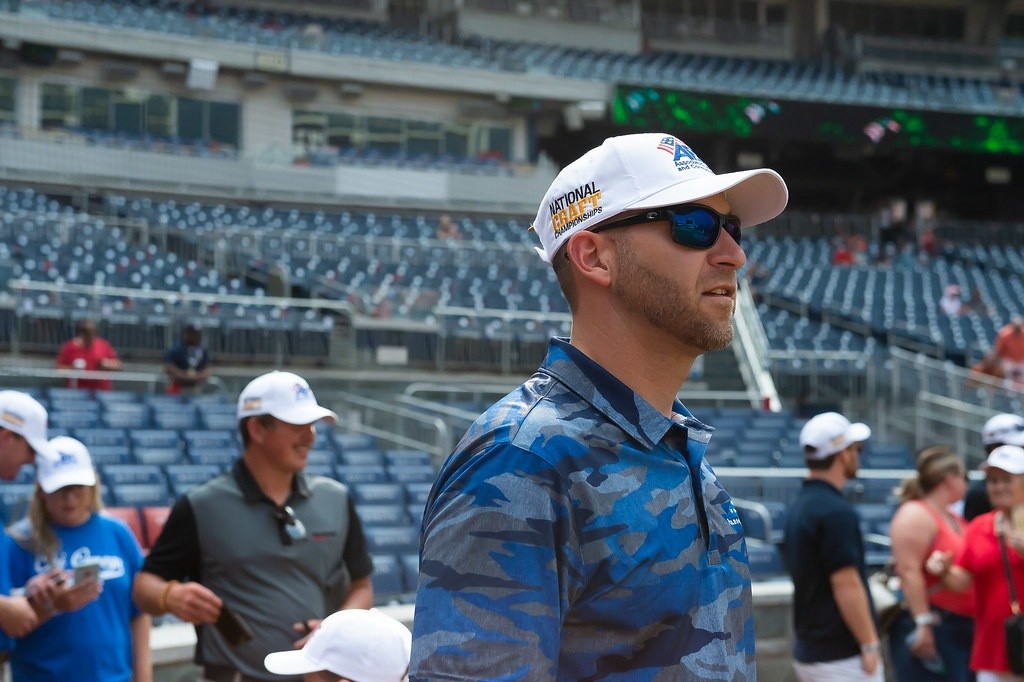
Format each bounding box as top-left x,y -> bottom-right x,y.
564,203 -> 742,261
849,444 -> 864,453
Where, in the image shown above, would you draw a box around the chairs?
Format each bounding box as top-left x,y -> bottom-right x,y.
0,0 -> 1024,607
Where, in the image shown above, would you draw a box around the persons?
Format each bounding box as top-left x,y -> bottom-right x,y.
131,369 -> 372,682
54,317 -> 120,390
0,390 -> 152,682
164,318 -> 210,395
437,215 -> 463,241
968,315 -> 1024,388
936,287 -> 986,313
832,199 -> 940,264
777,411 -> 1024,682
264,609 -> 412,682
410,134 -> 788,682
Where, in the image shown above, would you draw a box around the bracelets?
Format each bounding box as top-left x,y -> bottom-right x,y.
917,612 -> 931,627
158,580 -> 179,609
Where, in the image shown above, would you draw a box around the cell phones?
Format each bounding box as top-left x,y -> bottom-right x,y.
23,573 -> 67,601
75,563 -> 100,588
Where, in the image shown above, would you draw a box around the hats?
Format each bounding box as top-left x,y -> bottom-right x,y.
982,414 -> 1024,447
0,390 -> 63,462
237,370 -> 336,425
264,608 -> 412,682
799,411 -> 871,459
978,445 -> 1024,475
37,435 -> 96,494
533,133 -> 789,266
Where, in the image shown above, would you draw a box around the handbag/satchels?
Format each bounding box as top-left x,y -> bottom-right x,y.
869,571 -> 902,633
1006,614 -> 1024,676
